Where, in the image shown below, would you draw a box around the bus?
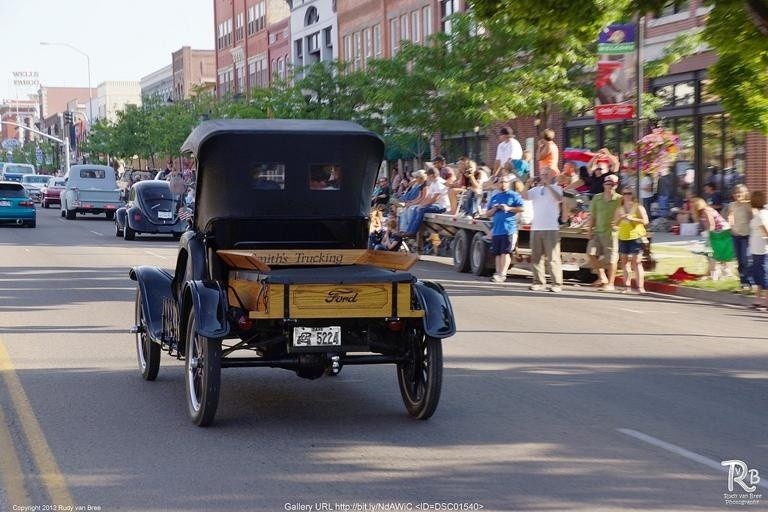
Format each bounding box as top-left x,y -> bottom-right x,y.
1,162 -> 36,181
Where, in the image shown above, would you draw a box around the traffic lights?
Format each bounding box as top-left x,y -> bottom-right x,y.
64,111 -> 74,126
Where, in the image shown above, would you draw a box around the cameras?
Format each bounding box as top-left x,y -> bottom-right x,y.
464,168 -> 473,174
533,176 -> 541,182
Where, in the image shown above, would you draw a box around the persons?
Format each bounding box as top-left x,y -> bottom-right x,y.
110,125 -> 768,315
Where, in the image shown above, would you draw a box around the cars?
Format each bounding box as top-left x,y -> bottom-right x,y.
116,178 -> 192,241
674,161 -> 718,189
20,175 -> 55,203
40,178 -> 68,208
0,181 -> 36,229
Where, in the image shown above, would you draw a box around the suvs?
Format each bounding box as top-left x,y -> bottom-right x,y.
128,117 -> 457,430
59,164 -> 125,220
116,169 -> 158,199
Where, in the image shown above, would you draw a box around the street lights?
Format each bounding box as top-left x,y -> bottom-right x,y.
39,41 -> 93,128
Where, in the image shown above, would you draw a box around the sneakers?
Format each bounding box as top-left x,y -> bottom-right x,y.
490,272 -> 561,293
